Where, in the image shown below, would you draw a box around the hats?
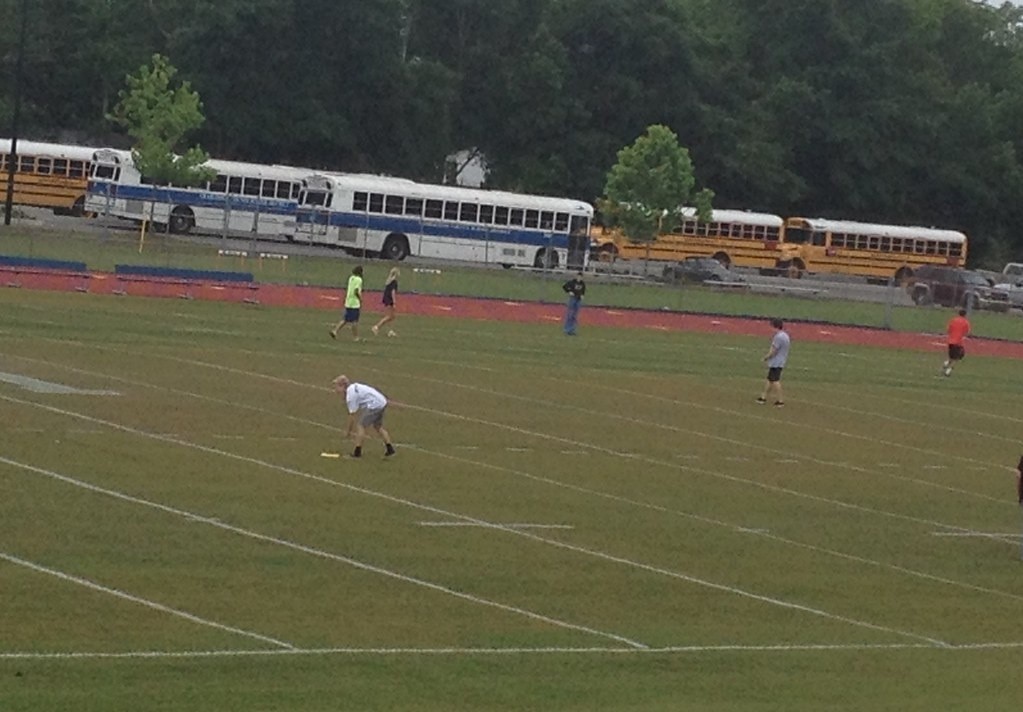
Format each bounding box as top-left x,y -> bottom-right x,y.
333,375 -> 348,383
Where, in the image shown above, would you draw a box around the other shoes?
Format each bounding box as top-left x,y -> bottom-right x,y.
943,361 -> 947,369
388,333 -> 396,337
347,454 -> 361,461
353,338 -> 359,342
381,450 -> 396,459
946,371 -> 951,376
330,330 -> 338,340
372,327 -> 378,335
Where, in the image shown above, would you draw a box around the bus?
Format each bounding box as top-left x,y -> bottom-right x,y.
761,217 -> 967,285
0,139 -> 106,216
294,175 -> 595,271
83,148 -> 311,235
581,200 -> 784,271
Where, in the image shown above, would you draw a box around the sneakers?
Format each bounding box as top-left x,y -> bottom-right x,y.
773,401 -> 784,408
755,397 -> 766,404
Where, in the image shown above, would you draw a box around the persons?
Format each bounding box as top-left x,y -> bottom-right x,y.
328,265 -> 367,341
331,375 -> 397,460
942,310 -> 971,377
372,267 -> 401,338
1015,455 -> 1023,507
756,318 -> 790,407
561,272 -> 585,336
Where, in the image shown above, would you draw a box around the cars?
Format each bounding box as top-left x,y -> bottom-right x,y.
913,262 -> 1023,312
663,256 -> 745,285
